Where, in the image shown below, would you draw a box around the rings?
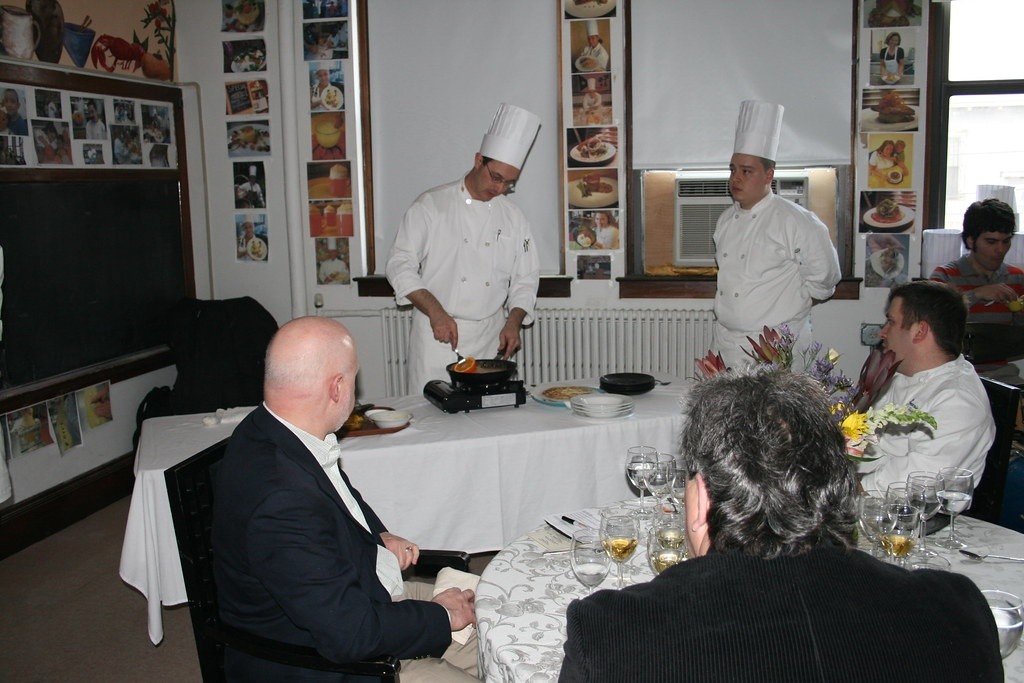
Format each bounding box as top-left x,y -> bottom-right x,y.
405,546 -> 412,552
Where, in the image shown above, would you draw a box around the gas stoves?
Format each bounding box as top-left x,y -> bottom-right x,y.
423,379 -> 526,414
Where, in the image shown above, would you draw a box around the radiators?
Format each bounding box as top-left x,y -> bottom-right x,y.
379,305 -> 716,398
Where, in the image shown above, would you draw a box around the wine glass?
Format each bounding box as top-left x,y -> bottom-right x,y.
571,446 -> 973,601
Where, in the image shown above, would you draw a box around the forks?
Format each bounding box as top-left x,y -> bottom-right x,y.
449,340 -> 467,366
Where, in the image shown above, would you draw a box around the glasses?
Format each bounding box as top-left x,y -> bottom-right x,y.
485,160 -> 516,189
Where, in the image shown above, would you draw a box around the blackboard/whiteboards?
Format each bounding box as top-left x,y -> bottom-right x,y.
0,166 -> 198,418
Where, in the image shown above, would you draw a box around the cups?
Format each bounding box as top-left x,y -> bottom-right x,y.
980,590 -> 1024,659
316,125 -> 340,148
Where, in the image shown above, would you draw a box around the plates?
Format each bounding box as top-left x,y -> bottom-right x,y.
600,372 -> 655,396
571,393 -> 635,422
231,50 -> 266,72
570,142 -> 616,162
227,124 -> 270,157
246,238 -> 267,260
565,0 -> 616,17
864,205 -> 914,227
569,177 -> 618,208
531,383 -> 605,406
321,87 -> 343,111
575,57 -> 600,71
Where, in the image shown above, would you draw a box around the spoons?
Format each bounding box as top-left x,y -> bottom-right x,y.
655,380 -> 671,385
959,550 -> 1024,561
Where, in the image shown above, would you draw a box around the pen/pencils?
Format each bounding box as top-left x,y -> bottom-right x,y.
562,516 -> 587,528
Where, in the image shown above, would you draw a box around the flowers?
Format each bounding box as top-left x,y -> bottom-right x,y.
686,322 -> 938,457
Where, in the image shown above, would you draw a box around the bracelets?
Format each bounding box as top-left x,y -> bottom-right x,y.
966,289 -> 981,304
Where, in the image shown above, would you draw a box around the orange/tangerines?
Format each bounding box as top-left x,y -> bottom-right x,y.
454,356 -> 476,373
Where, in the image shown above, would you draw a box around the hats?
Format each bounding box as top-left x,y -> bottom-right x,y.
327,239 -> 337,249
249,166 -> 256,176
242,214 -> 253,225
479,102 -> 539,171
586,21 -> 599,36
733,99 -> 785,161
315,62 -> 331,73
587,77 -> 596,90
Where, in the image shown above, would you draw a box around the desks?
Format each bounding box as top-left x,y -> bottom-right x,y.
116,373 -> 692,644
475,496 -> 1024,683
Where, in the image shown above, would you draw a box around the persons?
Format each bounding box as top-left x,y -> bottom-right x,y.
311,62 -> 333,110
319,237 -> 348,285
869,140 -> 905,179
0,88 -> 136,164
712,100 -> 842,378
583,78 -> 602,109
591,210 -> 619,249
558,366 -> 1006,683
580,21 -> 609,70
856,198 -> 1024,515
386,103 -> 540,397
209,316 -> 482,683
238,166 -> 266,258
880,32 -> 904,80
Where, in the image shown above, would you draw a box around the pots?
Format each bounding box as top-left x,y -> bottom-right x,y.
446,346 -> 517,385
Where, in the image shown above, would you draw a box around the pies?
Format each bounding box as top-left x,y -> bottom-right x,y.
542,387 -> 591,399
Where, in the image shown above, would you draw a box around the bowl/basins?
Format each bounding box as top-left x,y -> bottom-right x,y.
370,410 -> 412,428
883,76 -> 899,84
888,170 -> 902,182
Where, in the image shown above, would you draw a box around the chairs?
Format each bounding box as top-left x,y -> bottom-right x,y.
131,297 -> 280,457
959,374 -> 1021,527
162,436 -> 471,683
